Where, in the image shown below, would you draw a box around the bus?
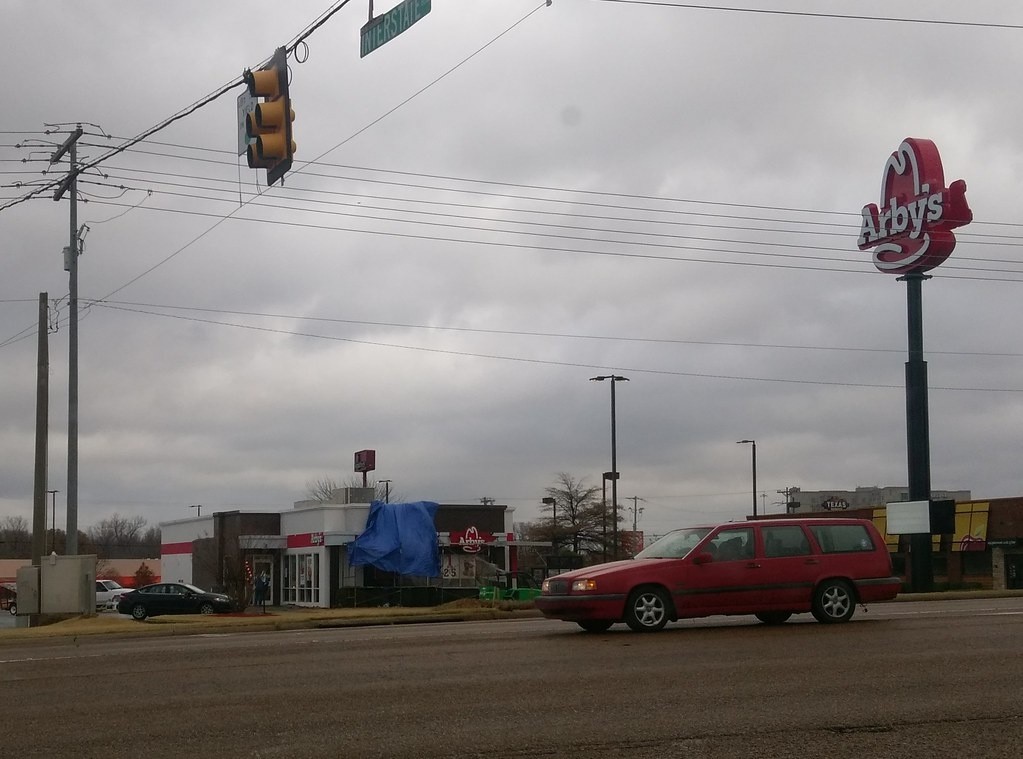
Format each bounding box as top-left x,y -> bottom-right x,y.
116,583 -> 233,620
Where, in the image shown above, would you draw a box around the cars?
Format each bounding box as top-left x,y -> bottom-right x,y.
96,579 -> 135,606
536,518 -> 906,632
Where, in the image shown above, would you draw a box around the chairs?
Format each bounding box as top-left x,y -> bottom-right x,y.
701,537 -> 736,562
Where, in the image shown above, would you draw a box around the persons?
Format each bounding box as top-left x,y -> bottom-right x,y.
256,570 -> 268,613
718,541 -> 738,560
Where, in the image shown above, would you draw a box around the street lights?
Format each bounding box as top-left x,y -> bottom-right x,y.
603,471 -> 620,561
735,438 -> 758,520
589,374 -> 630,560
47,490 -> 58,556
188,504 -> 202,517
541,496 -> 557,544
